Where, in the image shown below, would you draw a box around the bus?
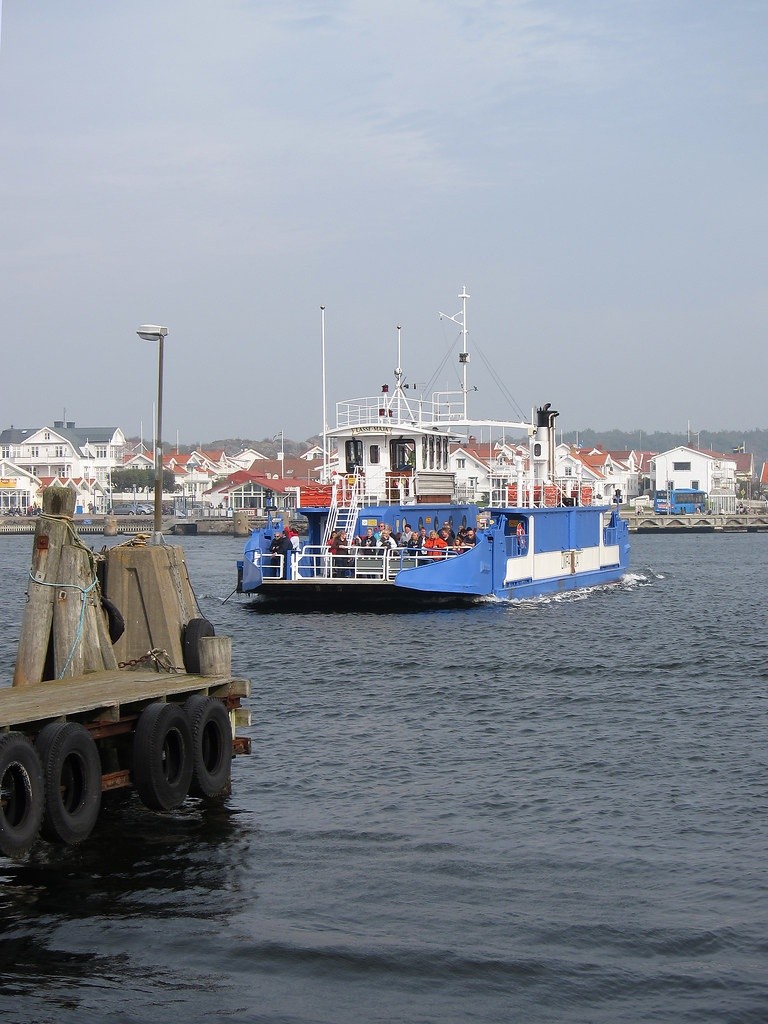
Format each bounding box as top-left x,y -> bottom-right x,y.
653,488 -> 709,515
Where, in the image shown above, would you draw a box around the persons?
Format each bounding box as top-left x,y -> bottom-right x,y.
16,506 -> 21,513
9,506 -> 14,513
209,503 -> 214,509
28,506 -> 33,514
634,506 -> 645,516
37,506 -> 41,512
289,528 -> 303,579
350,522 -> 399,556
331,471 -> 343,488
270,530 -> 293,577
408,521 -> 455,566
218,503 -> 223,508
328,530 -> 348,578
455,526 -> 475,555
87,501 -> 94,510
400,524 -> 413,543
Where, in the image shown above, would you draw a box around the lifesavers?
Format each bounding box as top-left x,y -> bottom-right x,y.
181,616 -> 217,674
35,723 -> 105,845
130,701 -> 197,812
0,728 -> 46,856
182,695 -> 235,799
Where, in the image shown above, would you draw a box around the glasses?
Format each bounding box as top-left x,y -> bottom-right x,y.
468,534 -> 473,536
378,526 -> 382,527
275,536 -> 279,537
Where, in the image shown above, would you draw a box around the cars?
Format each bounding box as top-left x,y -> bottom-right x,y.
107,503 -> 209,515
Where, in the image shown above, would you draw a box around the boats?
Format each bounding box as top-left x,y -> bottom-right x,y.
220,281 -> 634,608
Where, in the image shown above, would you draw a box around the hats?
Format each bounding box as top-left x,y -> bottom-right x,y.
332,471 -> 337,475
